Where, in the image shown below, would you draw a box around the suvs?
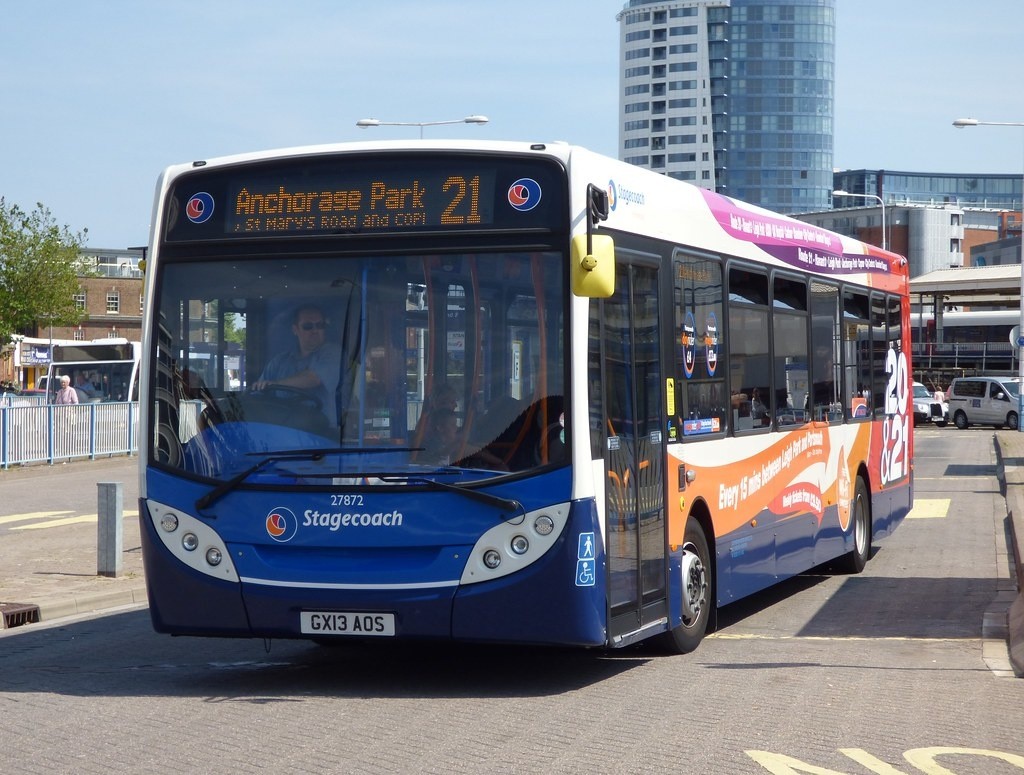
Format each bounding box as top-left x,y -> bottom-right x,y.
912,380 -> 947,427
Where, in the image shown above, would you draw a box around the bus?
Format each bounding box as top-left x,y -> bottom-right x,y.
135,137 -> 916,655
45,337 -> 230,449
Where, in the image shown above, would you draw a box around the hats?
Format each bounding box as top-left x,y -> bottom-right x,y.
753,387 -> 760,393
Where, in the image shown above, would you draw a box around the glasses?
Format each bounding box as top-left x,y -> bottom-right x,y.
296,322 -> 326,331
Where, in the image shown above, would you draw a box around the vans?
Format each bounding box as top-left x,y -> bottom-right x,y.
948,377 -> 1021,432
35,376 -> 64,394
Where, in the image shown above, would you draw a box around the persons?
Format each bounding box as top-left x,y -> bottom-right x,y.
752,376 -> 875,419
0,373 -> 123,432
253,302 -> 354,430
422,380 -> 511,477
934,386 -> 951,403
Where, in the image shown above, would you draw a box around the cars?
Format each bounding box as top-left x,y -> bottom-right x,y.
16,388 -> 52,403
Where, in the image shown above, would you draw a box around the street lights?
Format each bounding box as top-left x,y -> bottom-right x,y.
354,115 -> 492,426
831,190 -> 886,252
